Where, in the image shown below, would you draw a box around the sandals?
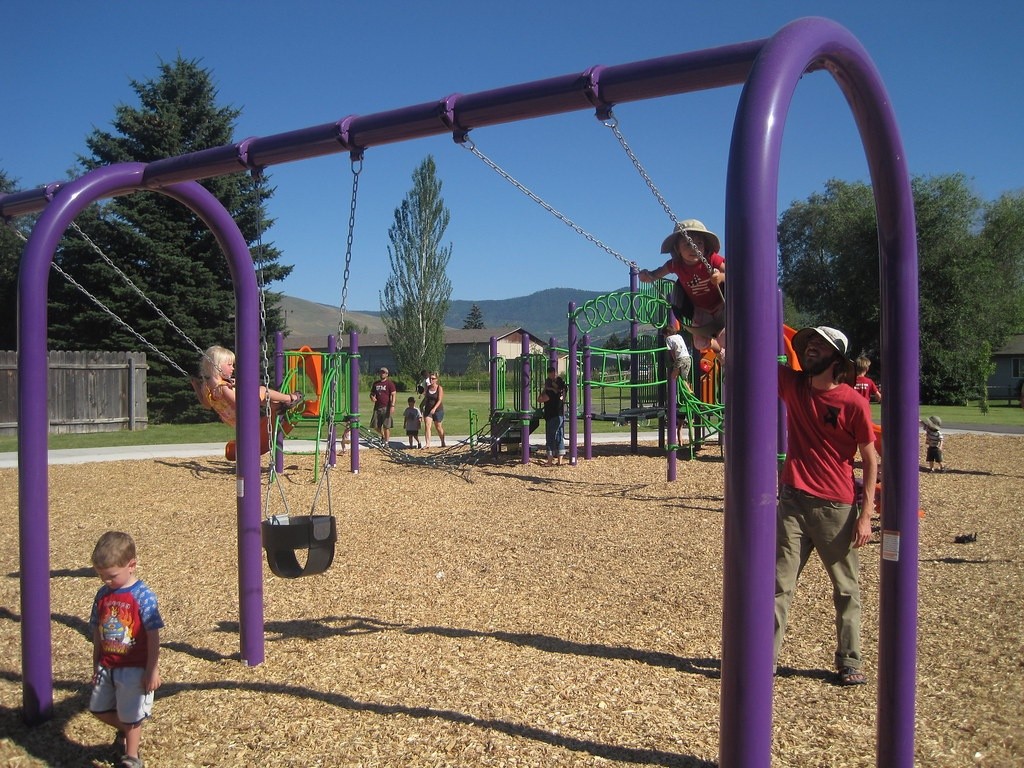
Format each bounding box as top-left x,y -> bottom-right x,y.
112,730 -> 127,755
281,391 -> 304,409
838,666 -> 867,685
119,753 -> 141,768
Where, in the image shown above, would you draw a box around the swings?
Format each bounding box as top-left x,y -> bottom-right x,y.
455,116 -> 737,340
245,159 -> 373,580
7,206 -> 274,431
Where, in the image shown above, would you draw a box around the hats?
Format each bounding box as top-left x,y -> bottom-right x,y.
381,367 -> 389,374
791,325 -> 856,387
660,219 -> 720,253
922,416 -> 941,431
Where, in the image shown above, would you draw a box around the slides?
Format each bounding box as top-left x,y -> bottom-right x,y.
224,398 -> 306,464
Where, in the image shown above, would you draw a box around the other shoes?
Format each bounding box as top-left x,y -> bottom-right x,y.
681,391 -> 695,399
541,462 -> 553,467
418,443 -> 421,448
384,443 -> 389,450
421,446 -> 429,450
928,468 -> 935,472
555,462 -> 562,466
442,442 -> 445,447
940,465 -> 946,474
717,348 -> 725,364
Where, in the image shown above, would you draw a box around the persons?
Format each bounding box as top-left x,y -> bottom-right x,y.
201,346 -> 301,427
638,219 -> 726,364
403,397 -> 422,449
369,367 -> 396,444
663,325 -> 692,393
537,367 -> 567,467
89,531 -> 164,768
420,370 -> 445,450
851,356 -> 882,404
919,416 -> 946,472
711,326 -> 877,685
677,414 -> 685,446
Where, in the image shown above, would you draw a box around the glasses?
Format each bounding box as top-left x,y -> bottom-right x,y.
430,378 -> 437,380
553,379 -> 558,385
